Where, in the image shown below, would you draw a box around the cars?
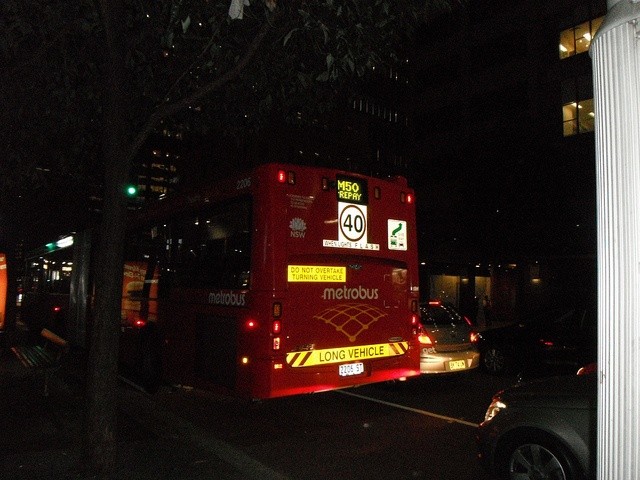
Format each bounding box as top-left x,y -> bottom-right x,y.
478,375 -> 596,479
420,299 -> 480,377
481,312 -> 595,374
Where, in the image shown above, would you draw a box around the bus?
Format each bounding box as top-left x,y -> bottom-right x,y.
23,232 -> 94,349
119,163 -> 422,398
